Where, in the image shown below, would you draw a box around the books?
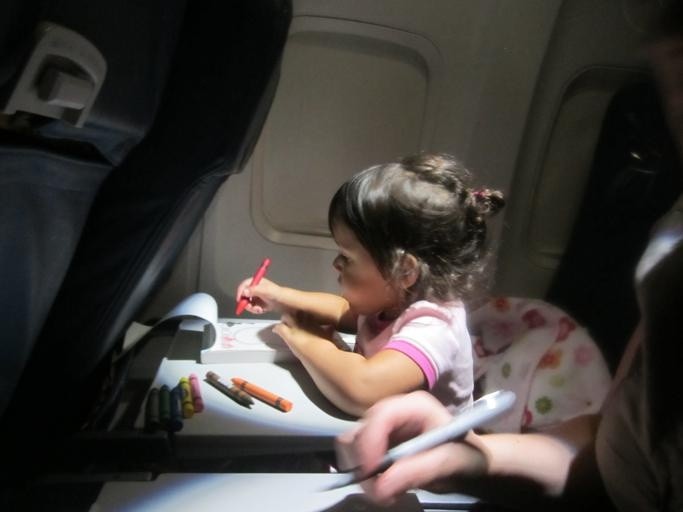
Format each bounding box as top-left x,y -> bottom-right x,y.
112,291 -> 350,365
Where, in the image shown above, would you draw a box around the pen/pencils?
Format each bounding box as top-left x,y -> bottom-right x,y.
147,373 -> 203,432
230,378 -> 293,412
206,371 -> 254,406
235,259 -> 271,318
319,389 -> 516,492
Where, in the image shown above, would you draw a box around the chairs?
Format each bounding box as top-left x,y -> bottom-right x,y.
0,4 -> 180,415
0,3 -> 297,449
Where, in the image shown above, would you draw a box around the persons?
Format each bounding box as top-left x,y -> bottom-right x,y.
328,193 -> 683,511
233,150 -> 480,421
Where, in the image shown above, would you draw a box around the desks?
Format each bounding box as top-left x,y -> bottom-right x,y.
90,472 -> 497,512
122,312 -> 378,462
